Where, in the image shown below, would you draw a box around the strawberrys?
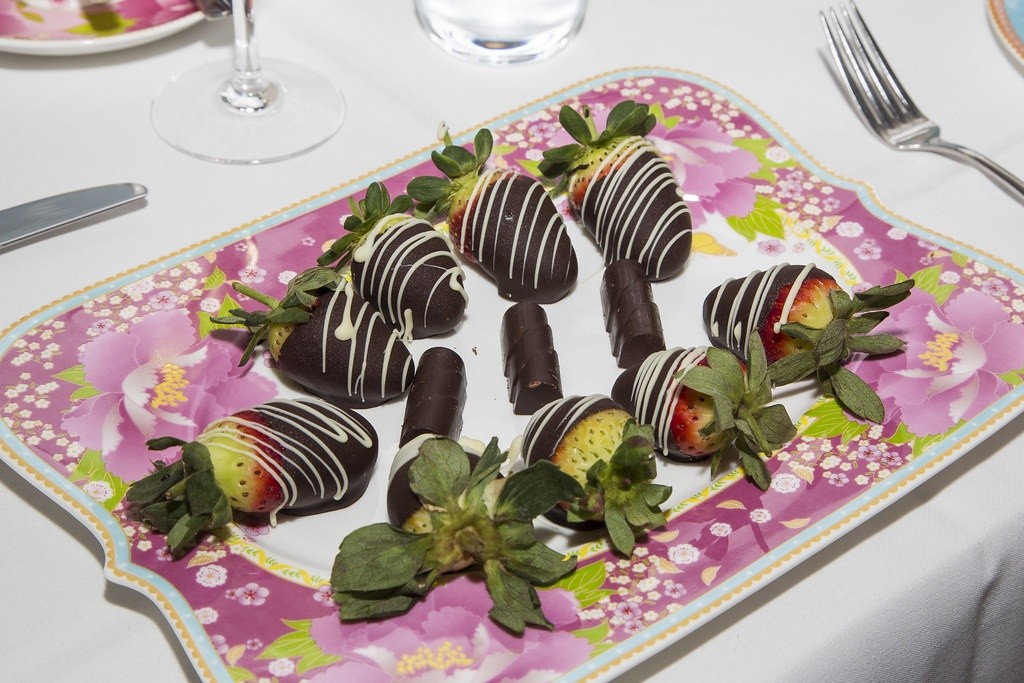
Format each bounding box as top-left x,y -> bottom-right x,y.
189,132 -> 847,573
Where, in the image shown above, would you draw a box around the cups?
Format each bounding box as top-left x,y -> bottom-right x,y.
413,0 -> 588,65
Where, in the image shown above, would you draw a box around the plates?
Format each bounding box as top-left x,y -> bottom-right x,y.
0,66 -> 1024,683
0,0 -> 206,57
987,0 -> 1024,66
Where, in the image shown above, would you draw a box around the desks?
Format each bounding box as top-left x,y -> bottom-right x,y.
0,0 -> 1024,683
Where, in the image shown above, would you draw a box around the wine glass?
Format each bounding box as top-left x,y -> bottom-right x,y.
151,0 -> 347,164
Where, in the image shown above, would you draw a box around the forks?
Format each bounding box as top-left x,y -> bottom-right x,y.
819,0 -> 1024,203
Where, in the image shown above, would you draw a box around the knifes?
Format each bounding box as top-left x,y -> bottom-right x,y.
0,182 -> 148,248
